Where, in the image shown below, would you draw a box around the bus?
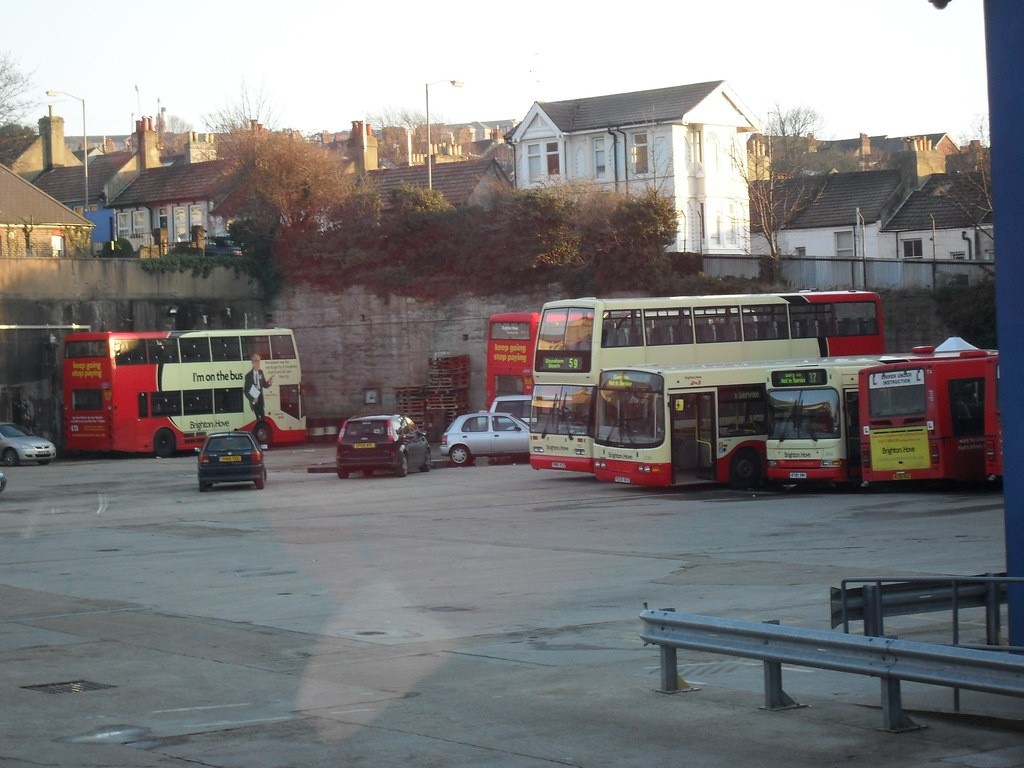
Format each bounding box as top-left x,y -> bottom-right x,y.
593,345 -> 1003,496
529,289 -> 887,474
60,328 -> 308,457
486,307 -> 759,413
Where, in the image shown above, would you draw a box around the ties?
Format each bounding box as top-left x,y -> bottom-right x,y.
256,373 -> 258,386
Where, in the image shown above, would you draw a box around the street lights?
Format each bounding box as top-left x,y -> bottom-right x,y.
425,78 -> 464,190
45,90 -> 89,212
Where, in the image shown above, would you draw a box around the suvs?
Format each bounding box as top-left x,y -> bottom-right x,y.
335,413 -> 432,479
439,411 -> 533,466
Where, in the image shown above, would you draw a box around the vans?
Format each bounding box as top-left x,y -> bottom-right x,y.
486,394 -> 538,429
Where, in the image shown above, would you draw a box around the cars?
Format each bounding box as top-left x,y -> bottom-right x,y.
194,431 -> 269,491
205,236 -> 243,257
0,423 -> 57,467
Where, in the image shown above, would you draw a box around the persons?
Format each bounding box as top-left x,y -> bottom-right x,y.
244,353 -> 273,422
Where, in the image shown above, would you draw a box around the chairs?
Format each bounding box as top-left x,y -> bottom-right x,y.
606,318 -> 878,347
728,420 -> 764,436
